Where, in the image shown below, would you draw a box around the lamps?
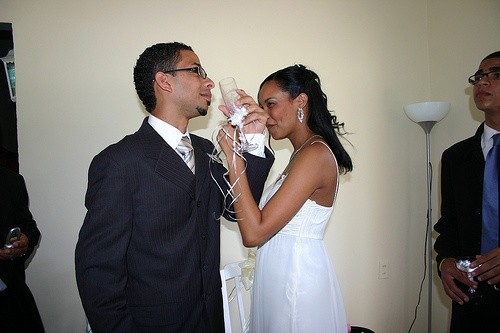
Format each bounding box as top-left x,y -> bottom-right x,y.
405,101 -> 453,333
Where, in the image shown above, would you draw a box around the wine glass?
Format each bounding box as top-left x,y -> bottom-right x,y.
207,125 -> 243,164
219,77 -> 259,153
455,252 -> 484,298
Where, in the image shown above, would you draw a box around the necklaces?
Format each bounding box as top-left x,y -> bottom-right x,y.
290,135 -> 323,161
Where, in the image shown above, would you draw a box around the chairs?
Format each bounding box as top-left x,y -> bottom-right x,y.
220,260 -> 252,333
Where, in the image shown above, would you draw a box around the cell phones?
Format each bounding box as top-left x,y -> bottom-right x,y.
5,227 -> 21,249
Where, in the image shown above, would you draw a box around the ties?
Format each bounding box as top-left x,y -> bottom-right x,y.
177,135 -> 196,175
481,133 -> 500,255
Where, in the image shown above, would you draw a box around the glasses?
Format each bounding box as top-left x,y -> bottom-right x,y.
468,70 -> 500,85
153,66 -> 207,79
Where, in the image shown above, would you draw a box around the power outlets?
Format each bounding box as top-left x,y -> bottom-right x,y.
378,263 -> 390,279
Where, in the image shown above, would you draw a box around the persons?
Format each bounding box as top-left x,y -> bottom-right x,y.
433,51 -> 500,333
216,65 -> 352,333
75,42 -> 275,333
0,172 -> 45,333
